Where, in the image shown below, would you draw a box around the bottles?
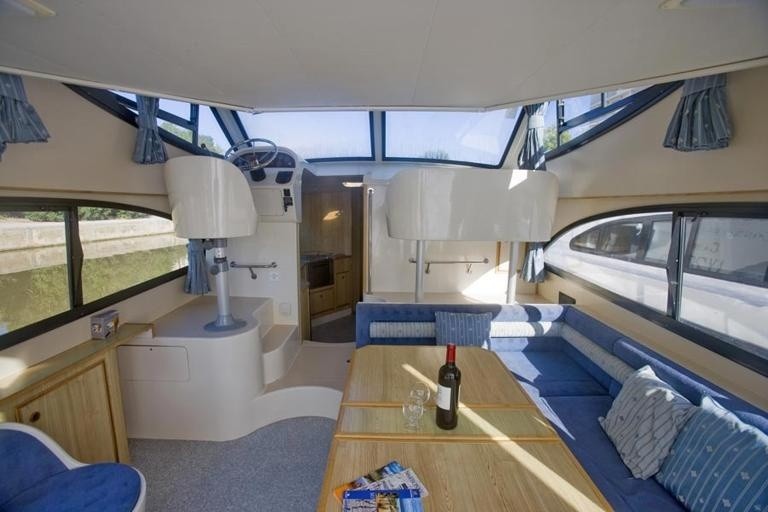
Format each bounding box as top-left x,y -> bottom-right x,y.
436,343 -> 461,430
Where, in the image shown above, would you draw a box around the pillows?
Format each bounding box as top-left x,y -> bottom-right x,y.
598,365 -> 699,481
434,311 -> 493,351
655,394 -> 768,512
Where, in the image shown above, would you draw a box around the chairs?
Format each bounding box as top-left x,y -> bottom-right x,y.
0,422 -> 147,512
163,155 -> 259,333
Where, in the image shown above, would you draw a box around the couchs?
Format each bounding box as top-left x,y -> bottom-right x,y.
355,301 -> 768,512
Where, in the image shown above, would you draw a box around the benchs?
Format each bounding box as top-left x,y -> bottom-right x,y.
386,165 -> 560,304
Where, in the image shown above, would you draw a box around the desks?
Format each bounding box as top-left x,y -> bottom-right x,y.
316,345 -> 616,512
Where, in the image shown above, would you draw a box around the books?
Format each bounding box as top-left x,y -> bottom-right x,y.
331,460 -> 429,512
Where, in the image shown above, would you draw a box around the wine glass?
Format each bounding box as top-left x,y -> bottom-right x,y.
410,382 -> 430,412
402,397 -> 424,430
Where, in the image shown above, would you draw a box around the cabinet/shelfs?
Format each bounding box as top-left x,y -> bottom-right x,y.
333,256 -> 354,312
0,322 -> 155,466
310,284 -> 336,320
